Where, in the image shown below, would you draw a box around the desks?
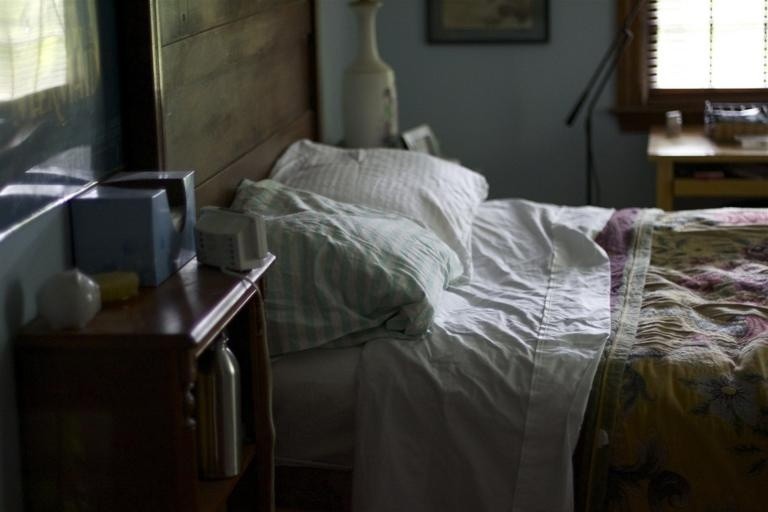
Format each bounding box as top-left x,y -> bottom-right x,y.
642,121 -> 767,210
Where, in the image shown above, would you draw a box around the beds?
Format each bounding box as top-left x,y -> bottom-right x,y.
158,0 -> 768,510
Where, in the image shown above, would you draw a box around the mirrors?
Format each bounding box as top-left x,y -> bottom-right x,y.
0,2 -> 129,245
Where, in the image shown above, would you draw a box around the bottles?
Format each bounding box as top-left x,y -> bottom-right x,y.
202,332 -> 244,479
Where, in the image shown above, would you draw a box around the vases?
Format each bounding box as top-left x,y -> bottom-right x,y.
340,0 -> 400,146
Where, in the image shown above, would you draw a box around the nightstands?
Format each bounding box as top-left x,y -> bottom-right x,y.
15,241 -> 278,512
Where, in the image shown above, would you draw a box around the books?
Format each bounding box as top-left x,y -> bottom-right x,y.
702,101 -> 768,149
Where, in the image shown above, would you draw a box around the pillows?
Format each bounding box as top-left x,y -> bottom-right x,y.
270,138 -> 488,280
234,178 -> 463,356
